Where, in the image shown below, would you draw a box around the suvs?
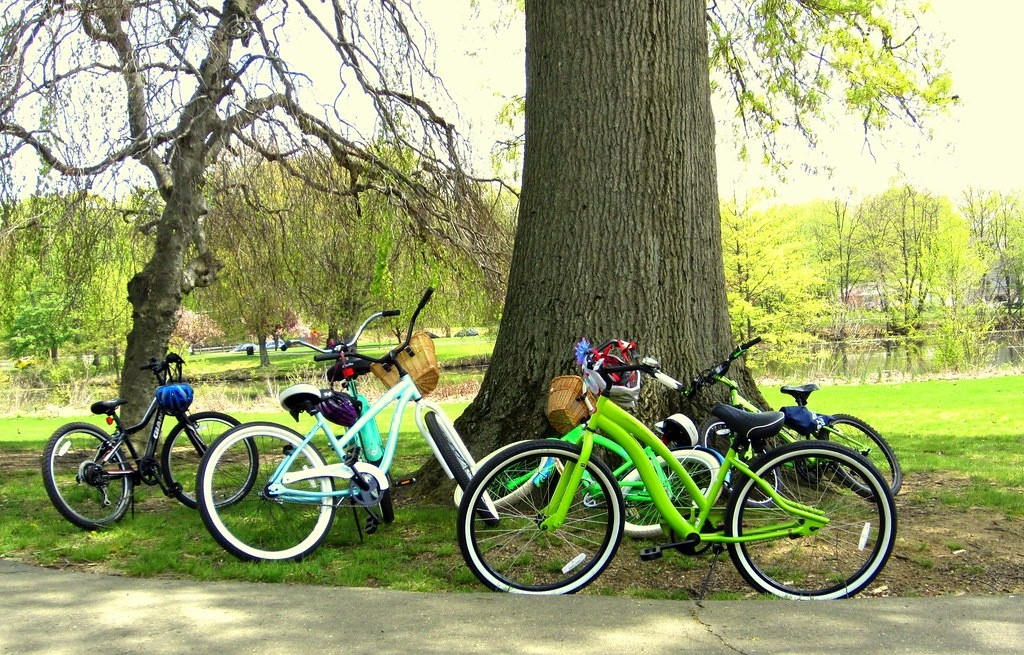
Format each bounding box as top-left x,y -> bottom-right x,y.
262,339 -> 284,349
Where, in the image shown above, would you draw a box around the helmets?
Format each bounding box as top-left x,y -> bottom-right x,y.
583,353 -> 642,406
156,383 -> 193,413
320,390 -> 360,428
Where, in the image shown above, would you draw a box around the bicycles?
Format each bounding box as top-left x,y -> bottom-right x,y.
452,335 -> 902,601
41,351 -> 258,531
195,285 -> 499,564
280,310 -> 417,525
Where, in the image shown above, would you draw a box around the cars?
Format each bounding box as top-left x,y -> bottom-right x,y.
233,343 -> 257,352
454,329 -> 478,337
424,332 -> 439,338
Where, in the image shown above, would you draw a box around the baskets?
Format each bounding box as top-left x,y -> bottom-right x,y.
545,375 -> 596,435
370,332 -> 438,401
